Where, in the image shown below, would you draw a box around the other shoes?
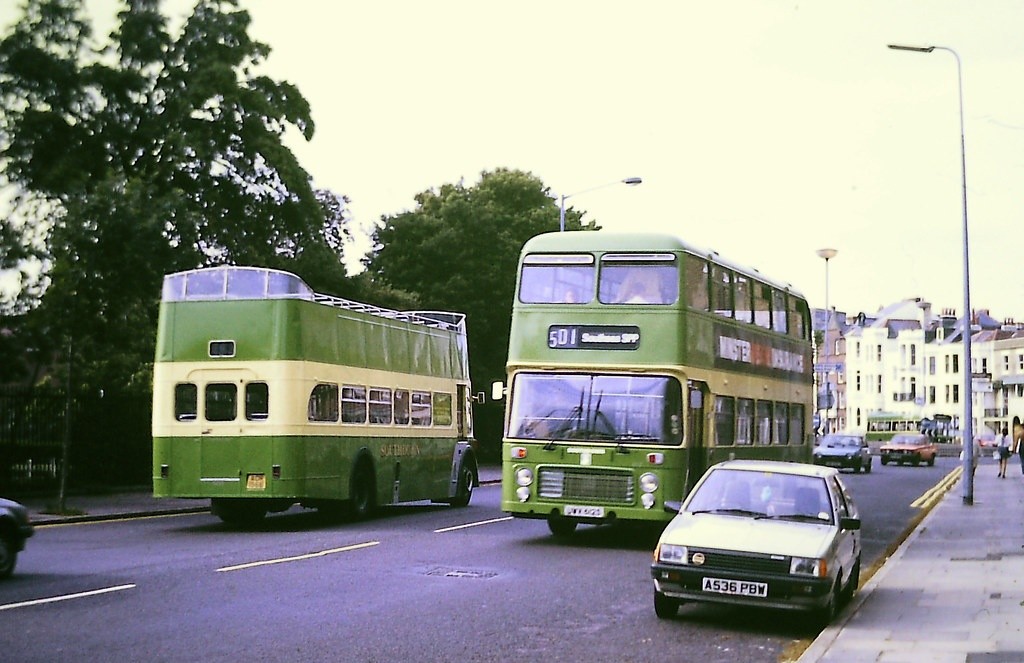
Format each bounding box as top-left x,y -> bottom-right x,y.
998,472 -> 1001,477
1003,475 -> 1005,478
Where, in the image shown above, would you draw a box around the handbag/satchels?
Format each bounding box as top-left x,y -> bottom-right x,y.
993,449 -> 1002,460
960,451 -> 964,460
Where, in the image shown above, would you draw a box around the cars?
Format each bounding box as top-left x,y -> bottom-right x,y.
0,498 -> 34,583
813,432 -> 873,474
972,433 -> 999,448
648,458 -> 861,634
879,432 -> 937,467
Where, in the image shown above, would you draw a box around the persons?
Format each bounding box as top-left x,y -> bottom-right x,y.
564,288 -> 579,303
1014,424 -> 1024,475
995,428 -> 1013,478
625,281 -> 648,304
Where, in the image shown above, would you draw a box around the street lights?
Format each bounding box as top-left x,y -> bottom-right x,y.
815,248 -> 839,437
559,177 -> 644,231
887,41 -> 974,505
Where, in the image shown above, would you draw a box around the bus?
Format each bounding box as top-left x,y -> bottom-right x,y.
492,231 -> 814,539
151,265 -> 486,525
865,412 -> 952,442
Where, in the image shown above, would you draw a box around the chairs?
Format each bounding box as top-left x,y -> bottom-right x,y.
794,487 -> 819,516
